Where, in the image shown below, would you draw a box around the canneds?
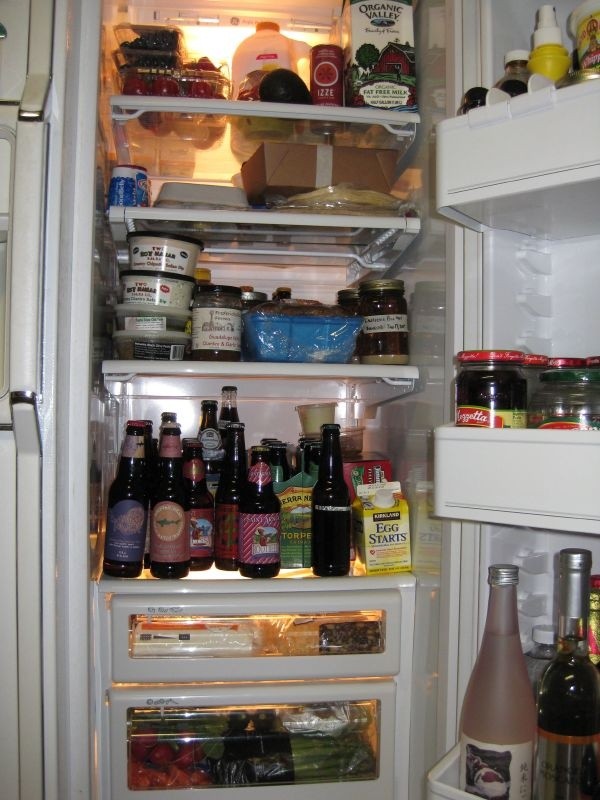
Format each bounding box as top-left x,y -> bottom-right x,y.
309,43 -> 343,106
190,284 -> 268,362
453,349 -> 600,432
338,279 -> 407,366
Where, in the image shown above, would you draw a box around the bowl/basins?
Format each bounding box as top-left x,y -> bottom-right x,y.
111,21 -> 230,151
110,229 -> 204,362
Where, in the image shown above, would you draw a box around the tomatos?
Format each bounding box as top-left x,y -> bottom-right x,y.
122,57 -> 227,100
124,728 -> 215,789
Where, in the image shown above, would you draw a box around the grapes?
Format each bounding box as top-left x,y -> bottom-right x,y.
122,30 -> 180,70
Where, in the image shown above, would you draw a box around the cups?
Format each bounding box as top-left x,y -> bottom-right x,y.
568,0 -> 600,81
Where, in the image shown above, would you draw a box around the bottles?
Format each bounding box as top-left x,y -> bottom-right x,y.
531,547 -> 600,800
587,574 -> 600,675
459,563 -> 537,800
525,4 -> 571,89
522,624 -> 554,707
230,20 -> 313,158
494,48 -> 531,98
454,349 -> 600,431
103,386 -> 352,578
190,267 -> 410,365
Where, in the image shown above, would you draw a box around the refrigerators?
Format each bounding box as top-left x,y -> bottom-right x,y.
0,0 -> 600,800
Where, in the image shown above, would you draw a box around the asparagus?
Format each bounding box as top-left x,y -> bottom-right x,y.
209,729 -> 374,782
